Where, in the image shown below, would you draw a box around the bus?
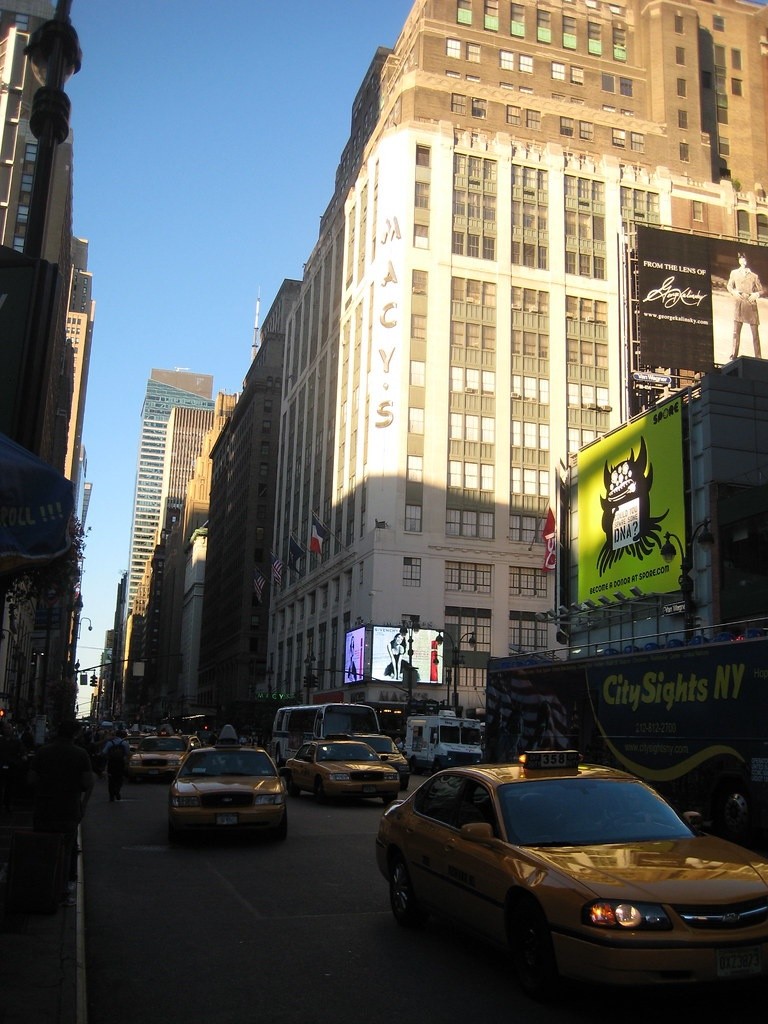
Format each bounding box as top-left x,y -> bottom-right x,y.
270,701 -> 381,768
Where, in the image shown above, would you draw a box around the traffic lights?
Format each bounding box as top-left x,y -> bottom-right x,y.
311,674 -> 318,688
94,675 -> 98,687
304,676 -> 311,687
90,675 -> 94,686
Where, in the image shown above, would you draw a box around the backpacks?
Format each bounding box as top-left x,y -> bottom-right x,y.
107,739 -> 126,762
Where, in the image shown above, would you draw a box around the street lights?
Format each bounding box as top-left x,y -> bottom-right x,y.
435,627 -> 477,718
399,617 -> 420,717
303,652 -> 316,705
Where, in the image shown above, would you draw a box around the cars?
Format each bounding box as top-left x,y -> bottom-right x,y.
327,732 -> 411,791
285,734 -> 399,807
168,724 -> 288,846
374,751 -> 768,1013
100,720 -> 201,783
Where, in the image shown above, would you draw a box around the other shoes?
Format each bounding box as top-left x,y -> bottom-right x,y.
110,797 -> 114,801
115,790 -> 121,800
58,896 -> 76,907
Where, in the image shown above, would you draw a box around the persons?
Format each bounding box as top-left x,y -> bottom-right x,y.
78,730 -> 130,801
201,729 -> 253,745
384,633 -> 420,680
27,728 -> 94,905
348,636 -> 357,682
726,252 -> 764,359
0,722 -> 34,811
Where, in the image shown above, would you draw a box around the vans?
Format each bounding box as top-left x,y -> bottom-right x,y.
404,710 -> 484,775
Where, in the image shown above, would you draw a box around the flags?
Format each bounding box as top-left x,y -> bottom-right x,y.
288,535 -> 304,569
542,508 -> 555,572
253,571 -> 265,603
311,514 -> 327,554
272,557 -> 283,585
